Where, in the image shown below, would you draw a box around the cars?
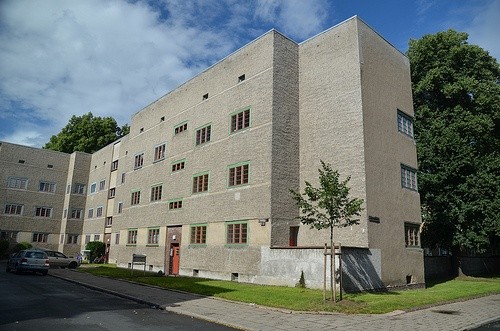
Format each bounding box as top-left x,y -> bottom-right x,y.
42,249 -> 81,270
7,249 -> 50,275
